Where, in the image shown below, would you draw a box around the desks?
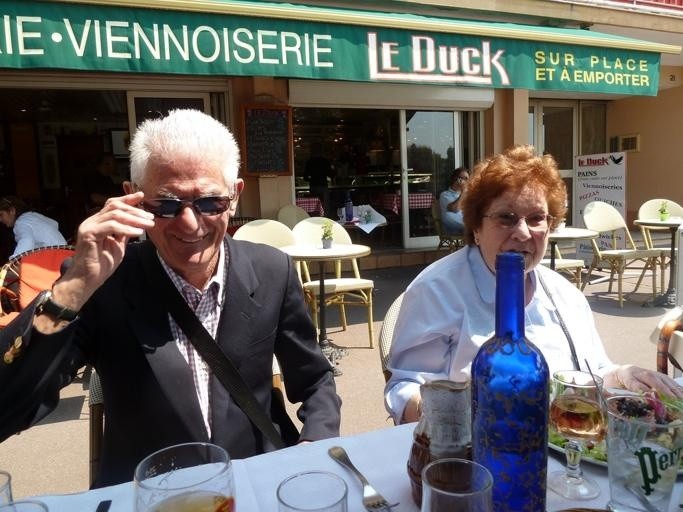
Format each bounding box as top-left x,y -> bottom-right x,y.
547,227 -> 598,273
631,217 -> 681,309
283,244 -> 371,361
377,192 -> 437,249
297,196 -> 325,218
342,221 -> 389,296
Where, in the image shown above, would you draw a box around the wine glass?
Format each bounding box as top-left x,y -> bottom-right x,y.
551,367 -> 602,501
337,208 -> 344,223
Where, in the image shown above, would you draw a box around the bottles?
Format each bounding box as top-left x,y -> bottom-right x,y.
473,248 -> 546,511
344,192 -> 353,221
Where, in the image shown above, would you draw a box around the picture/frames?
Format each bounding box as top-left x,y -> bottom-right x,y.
108,127 -> 130,161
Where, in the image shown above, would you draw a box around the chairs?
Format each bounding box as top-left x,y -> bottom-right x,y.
379,289 -> 406,428
233,220 -> 292,249
541,242 -> 583,292
432,200 -> 463,260
291,216 -> 375,349
227,217 -> 255,240
1,246 -> 78,327
83,355 -> 284,488
632,198 -> 681,301
277,200 -> 312,286
579,200 -> 661,310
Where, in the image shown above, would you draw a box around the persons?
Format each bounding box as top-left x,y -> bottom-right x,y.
383,145 -> 683,427
303,156 -> 336,212
440,167 -> 469,236
0,176 -> 114,261
0,109 -> 342,489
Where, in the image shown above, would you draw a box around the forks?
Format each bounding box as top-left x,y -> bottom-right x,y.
327,445 -> 403,510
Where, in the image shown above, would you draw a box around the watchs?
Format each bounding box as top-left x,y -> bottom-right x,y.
33,290 -> 79,323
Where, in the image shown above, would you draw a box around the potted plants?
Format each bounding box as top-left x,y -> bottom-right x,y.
319,218 -> 335,250
656,200 -> 670,221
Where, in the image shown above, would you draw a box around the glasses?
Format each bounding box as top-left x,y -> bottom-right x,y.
134,183 -> 237,220
481,210 -> 556,231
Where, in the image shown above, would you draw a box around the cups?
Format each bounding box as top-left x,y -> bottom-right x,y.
131,440 -> 235,511
605,399 -> 681,510
275,469 -> 349,510
0,473 -> 49,512
418,455 -> 496,511
